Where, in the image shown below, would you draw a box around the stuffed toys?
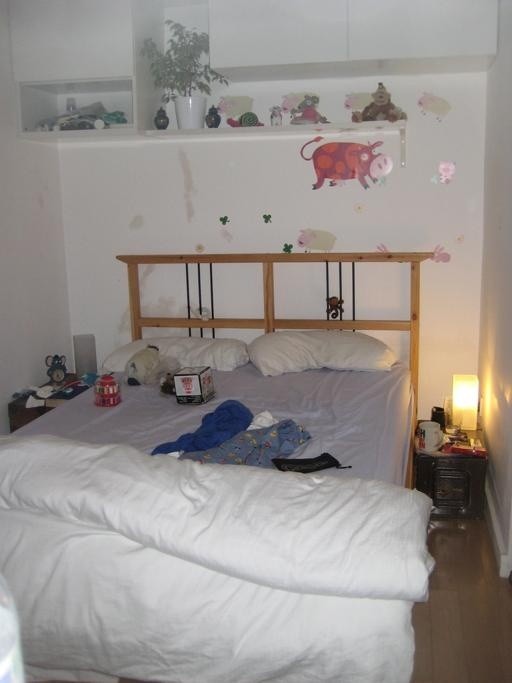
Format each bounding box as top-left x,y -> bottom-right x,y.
290,94 -> 331,125
351,82 -> 408,124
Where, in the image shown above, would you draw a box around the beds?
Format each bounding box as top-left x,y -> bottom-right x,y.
2,254 -> 422,682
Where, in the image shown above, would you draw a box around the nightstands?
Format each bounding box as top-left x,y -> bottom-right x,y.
410,425 -> 487,535
8,382 -> 54,432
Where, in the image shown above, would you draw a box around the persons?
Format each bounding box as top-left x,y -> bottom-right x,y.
269,105 -> 283,127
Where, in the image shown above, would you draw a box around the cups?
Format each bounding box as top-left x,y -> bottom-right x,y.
418,421 -> 445,450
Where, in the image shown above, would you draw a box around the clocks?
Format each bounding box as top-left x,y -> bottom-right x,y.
44,354 -> 67,383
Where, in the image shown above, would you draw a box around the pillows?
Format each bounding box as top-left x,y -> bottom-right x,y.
101,330 -> 398,376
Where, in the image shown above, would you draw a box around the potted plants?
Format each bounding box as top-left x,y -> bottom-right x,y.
139,19 -> 231,131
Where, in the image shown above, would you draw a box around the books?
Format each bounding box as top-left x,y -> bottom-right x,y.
44,385 -> 90,408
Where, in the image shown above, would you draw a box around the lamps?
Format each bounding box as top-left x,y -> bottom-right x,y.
453,375 -> 479,431
